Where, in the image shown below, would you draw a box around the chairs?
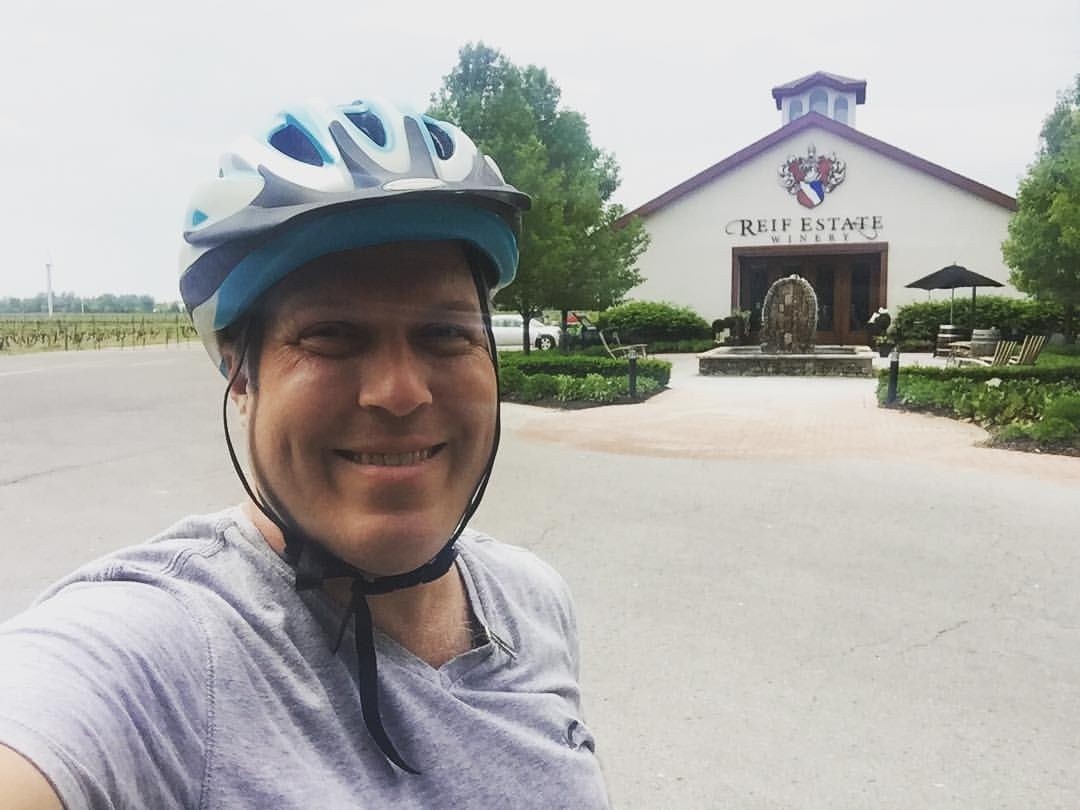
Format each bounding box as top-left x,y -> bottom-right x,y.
980,335 -> 1043,366
948,341 -> 1017,368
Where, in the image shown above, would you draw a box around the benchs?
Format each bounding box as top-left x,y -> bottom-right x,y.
598,326 -> 648,358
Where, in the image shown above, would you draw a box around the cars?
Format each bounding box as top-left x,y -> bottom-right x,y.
491,314 -> 562,351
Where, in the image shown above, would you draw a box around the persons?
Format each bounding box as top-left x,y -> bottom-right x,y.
1,95 -> 587,808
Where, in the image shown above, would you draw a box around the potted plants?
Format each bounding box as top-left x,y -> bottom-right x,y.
711,307 -> 752,346
869,307 -> 893,358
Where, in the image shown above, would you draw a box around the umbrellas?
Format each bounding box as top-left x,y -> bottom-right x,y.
905,265 -> 1004,325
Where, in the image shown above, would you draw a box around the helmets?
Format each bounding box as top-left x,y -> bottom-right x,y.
175,100 -> 533,378
